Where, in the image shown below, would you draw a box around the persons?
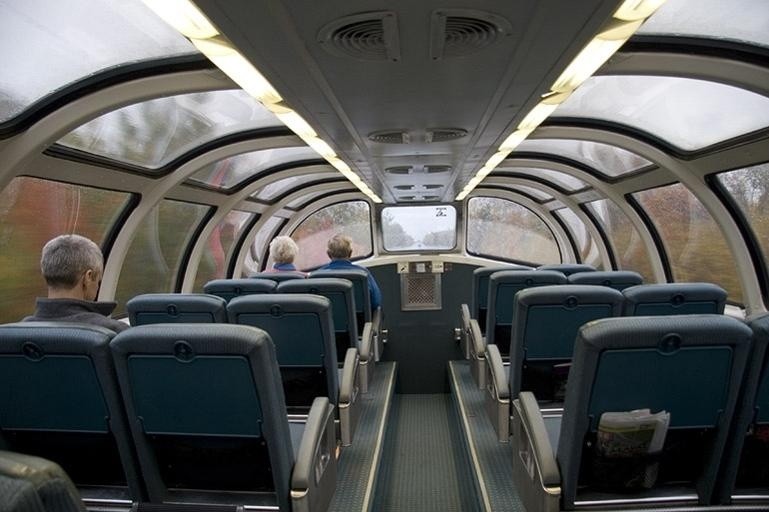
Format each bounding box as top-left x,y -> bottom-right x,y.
21,232 -> 130,334
261,235 -> 307,277
308,231 -> 381,310
